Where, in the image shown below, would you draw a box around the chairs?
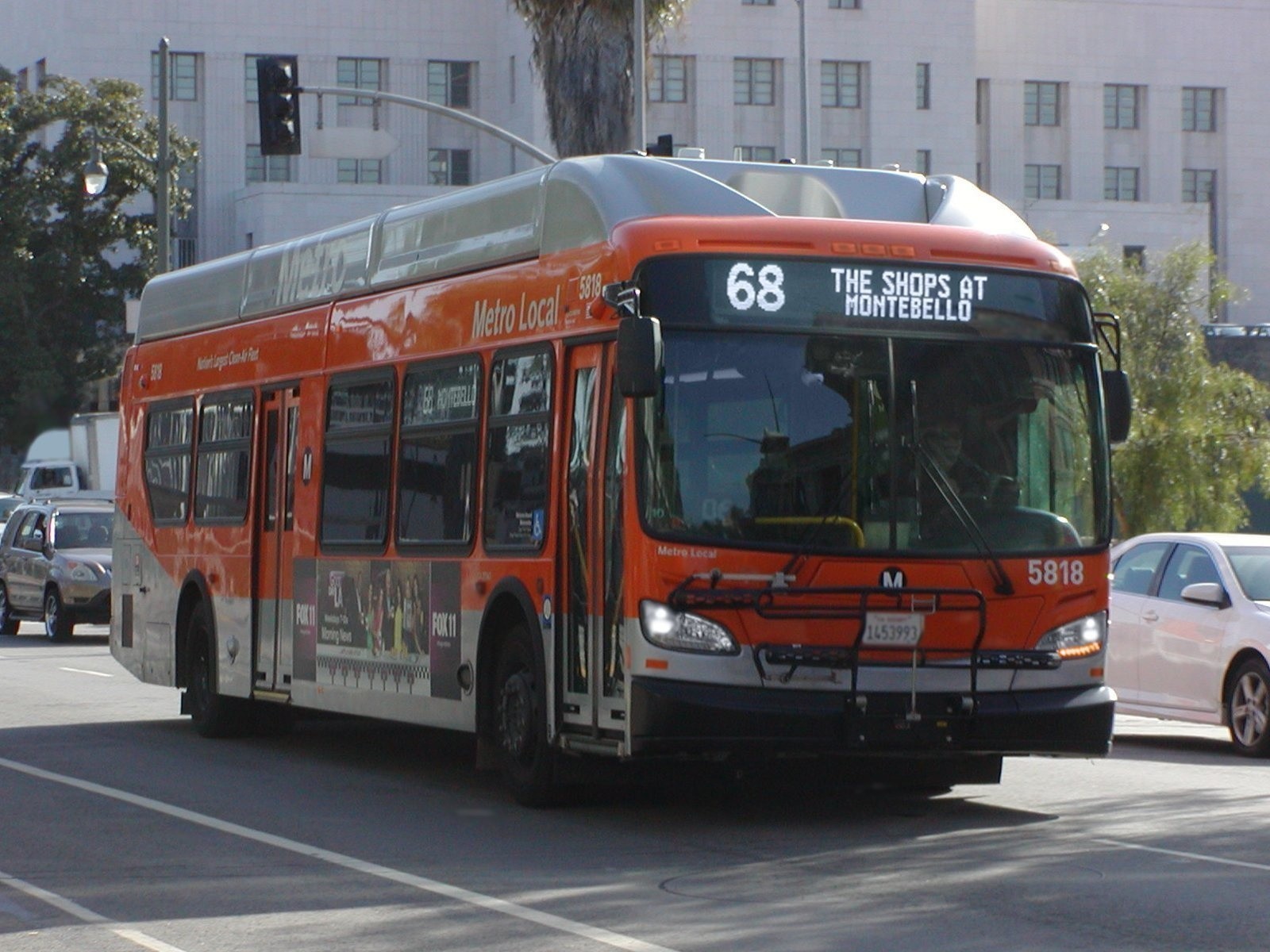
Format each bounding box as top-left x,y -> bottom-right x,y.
58,525 -> 79,546
1121,555 -> 1268,599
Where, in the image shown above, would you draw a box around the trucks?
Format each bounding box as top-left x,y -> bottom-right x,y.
10,411 -> 122,502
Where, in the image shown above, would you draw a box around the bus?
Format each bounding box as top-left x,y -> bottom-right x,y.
109,146 -> 1131,815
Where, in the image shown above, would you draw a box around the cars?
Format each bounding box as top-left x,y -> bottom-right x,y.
1101,531 -> 1270,759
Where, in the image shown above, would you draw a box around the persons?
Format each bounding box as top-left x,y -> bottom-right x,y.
94,524 -> 109,546
882,408 -> 986,516
59,524 -> 82,548
355,569 -> 426,664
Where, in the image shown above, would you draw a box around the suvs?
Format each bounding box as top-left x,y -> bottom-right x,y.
0,497 -> 116,644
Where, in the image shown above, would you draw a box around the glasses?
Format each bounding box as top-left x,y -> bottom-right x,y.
936,430 -> 962,440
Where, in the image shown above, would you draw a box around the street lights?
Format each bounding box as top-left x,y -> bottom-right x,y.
81,34 -> 183,279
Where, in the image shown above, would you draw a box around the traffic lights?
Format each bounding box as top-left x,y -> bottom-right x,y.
254,54 -> 302,156
